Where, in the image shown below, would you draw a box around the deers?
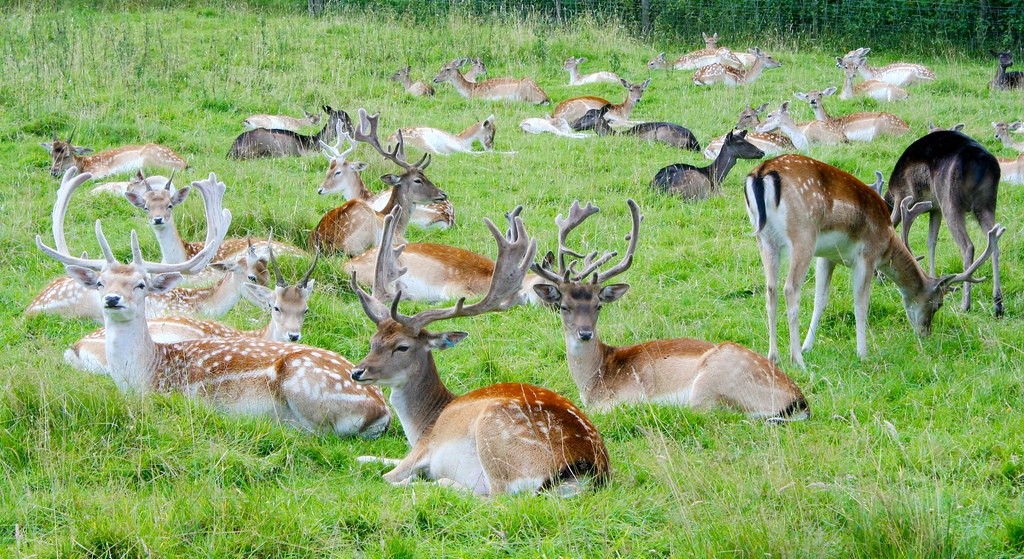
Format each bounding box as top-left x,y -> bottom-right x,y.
347,202 -> 613,500
24,31 -> 1020,442
